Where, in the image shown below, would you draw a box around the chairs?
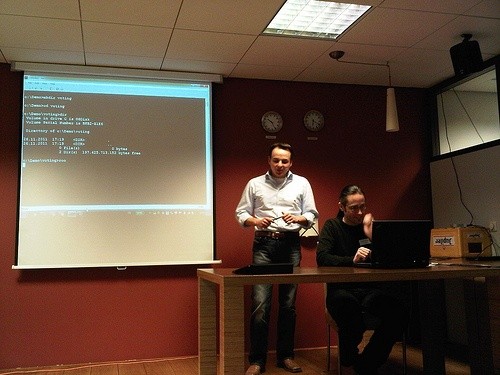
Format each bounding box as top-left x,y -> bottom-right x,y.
316,240 -> 411,375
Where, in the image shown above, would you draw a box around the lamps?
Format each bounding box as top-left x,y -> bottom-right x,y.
329,51 -> 399,132
450,34 -> 486,80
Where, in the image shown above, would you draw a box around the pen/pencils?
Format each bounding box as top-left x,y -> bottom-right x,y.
272,216 -> 284,220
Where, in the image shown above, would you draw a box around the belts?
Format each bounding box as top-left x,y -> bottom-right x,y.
255,231 -> 297,237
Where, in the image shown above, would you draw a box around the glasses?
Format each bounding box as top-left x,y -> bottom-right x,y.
345,205 -> 367,212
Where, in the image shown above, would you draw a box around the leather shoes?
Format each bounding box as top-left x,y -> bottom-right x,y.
278,356 -> 301,372
246,364 -> 261,375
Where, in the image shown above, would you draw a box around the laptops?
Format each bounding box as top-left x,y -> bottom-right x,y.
354,220 -> 432,269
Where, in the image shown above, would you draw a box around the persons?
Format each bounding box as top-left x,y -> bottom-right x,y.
316,184 -> 408,375
235,144 -> 320,375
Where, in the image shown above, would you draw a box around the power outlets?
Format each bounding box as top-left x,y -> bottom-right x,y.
489,221 -> 497,232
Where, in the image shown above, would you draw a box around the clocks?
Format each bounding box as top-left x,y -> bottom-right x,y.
303,110 -> 325,132
261,111 -> 283,134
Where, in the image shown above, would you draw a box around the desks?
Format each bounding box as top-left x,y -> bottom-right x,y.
196,258 -> 500,375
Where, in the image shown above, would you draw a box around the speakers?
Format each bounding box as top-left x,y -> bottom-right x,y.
450,40 -> 483,76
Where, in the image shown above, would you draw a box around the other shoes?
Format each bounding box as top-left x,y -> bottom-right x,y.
341,366 -> 357,375
